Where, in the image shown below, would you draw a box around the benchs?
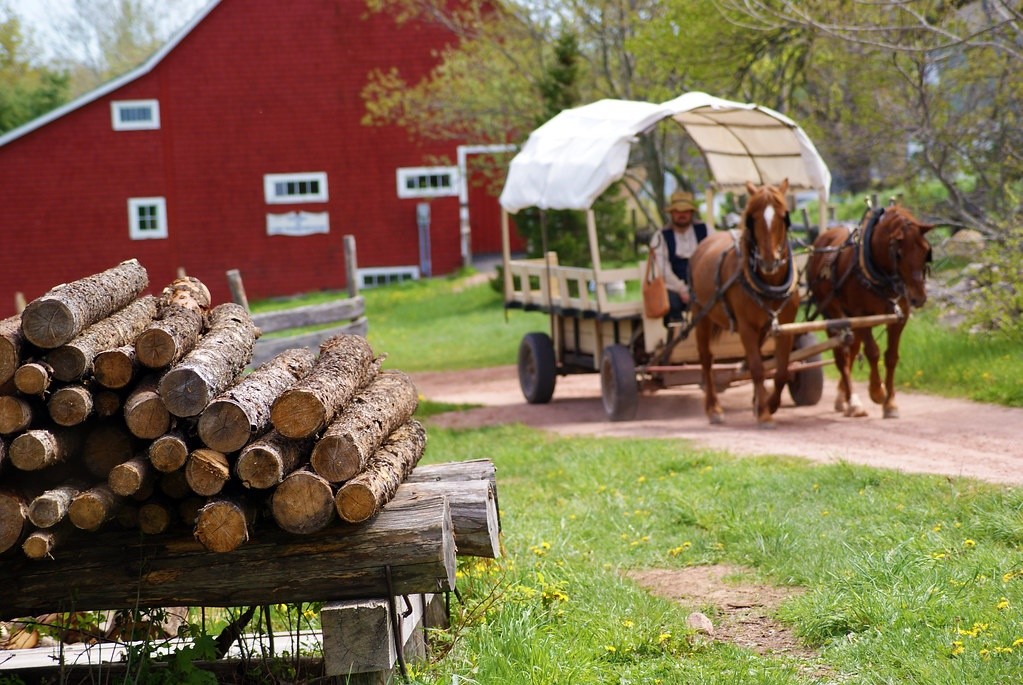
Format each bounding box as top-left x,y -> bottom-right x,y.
634,259 -> 777,358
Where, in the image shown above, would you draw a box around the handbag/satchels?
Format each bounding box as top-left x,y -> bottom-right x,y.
643,248 -> 670,317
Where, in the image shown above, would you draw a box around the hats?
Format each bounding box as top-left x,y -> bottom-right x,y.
664,191 -> 697,213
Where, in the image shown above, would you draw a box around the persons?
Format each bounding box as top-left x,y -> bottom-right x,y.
651,191 -> 718,328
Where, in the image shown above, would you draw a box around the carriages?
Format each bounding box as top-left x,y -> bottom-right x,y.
499,91 -> 936,427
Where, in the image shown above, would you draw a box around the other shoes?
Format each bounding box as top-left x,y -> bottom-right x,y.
664,309 -> 683,329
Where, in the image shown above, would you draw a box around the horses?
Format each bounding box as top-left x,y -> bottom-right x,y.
685,178 -> 801,430
806,205 -> 934,419
